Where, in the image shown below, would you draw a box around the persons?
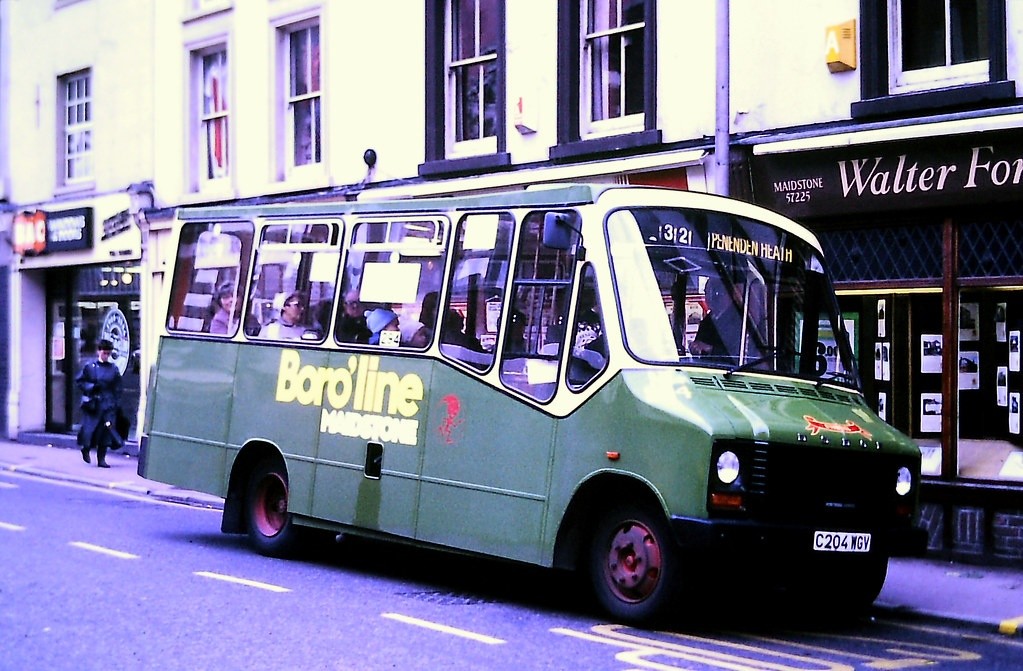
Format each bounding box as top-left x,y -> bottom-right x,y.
259,292 -> 318,339
363,308 -> 401,345
74,339 -> 122,468
410,292 -> 468,348
209,280 -> 262,337
337,290 -> 373,344
689,279 -> 742,366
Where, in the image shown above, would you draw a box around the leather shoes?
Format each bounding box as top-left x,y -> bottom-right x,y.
99,461 -> 111,468
81,448 -> 91,463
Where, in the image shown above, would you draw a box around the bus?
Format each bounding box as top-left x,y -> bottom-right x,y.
133,182 -> 922,633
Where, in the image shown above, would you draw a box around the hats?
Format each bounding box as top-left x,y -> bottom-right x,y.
274,292 -> 294,313
365,309 -> 397,333
96,341 -> 114,351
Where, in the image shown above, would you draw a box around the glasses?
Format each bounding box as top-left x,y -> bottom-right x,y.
288,302 -> 306,310
345,300 -> 364,307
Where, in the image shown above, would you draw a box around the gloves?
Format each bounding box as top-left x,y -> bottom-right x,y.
94,384 -> 101,390
